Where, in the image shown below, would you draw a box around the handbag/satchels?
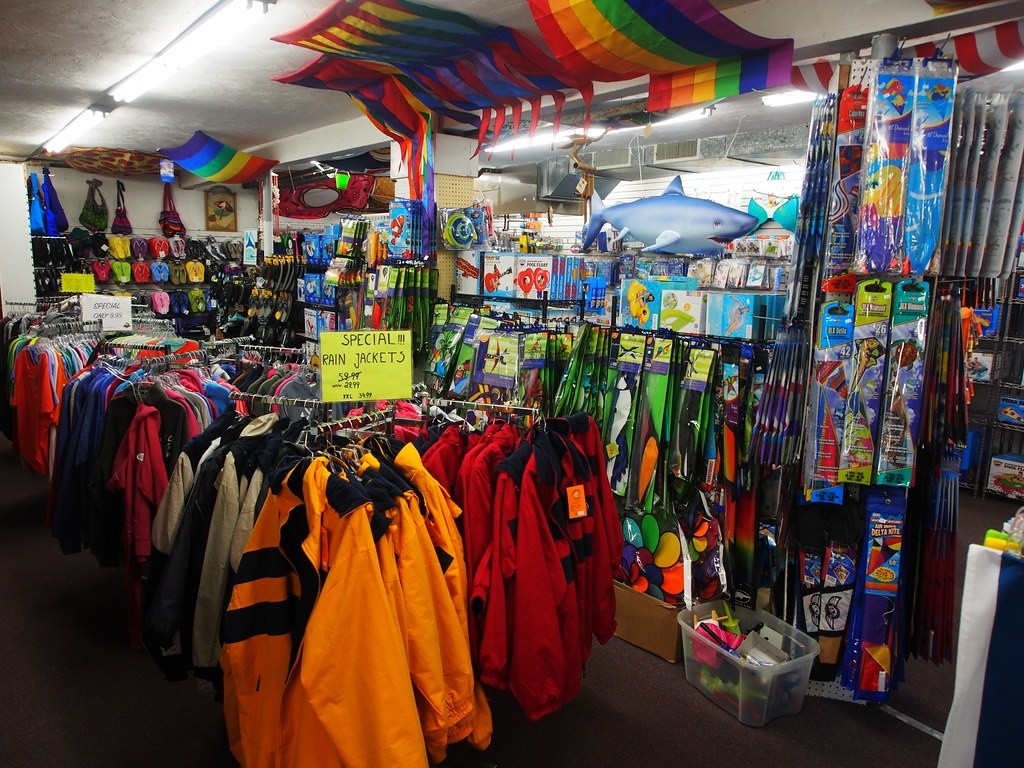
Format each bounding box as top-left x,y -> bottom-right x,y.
159,182 -> 186,238
27,167 -> 69,236
112,180 -> 132,235
79,178 -> 109,233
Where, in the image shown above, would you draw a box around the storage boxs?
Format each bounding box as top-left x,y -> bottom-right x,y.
615,576 -> 725,664
676,600 -> 819,727
987,455 -> 1024,499
454,252 -> 785,342
967,353 -> 994,383
296,274 -> 335,306
998,394 -> 1024,426
959,430 -> 981,470
302,225 -> 341,266
974,303 -> 999,336
303,308 -> 337,338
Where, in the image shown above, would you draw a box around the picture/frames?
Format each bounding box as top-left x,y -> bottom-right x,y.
203,185 -> 237,232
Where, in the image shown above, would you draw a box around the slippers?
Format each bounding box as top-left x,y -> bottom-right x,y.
32,228 -> 305,356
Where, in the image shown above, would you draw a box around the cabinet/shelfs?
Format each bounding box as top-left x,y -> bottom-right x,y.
959,259 -> 1024,503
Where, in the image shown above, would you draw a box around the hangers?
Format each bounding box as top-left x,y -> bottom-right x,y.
131,318 -> 176,342
4,296 -> 103,352
99,341 -> 213,387
425,396 -> 547,440
214,337 -> 316,380
219,391 -> 394,473
